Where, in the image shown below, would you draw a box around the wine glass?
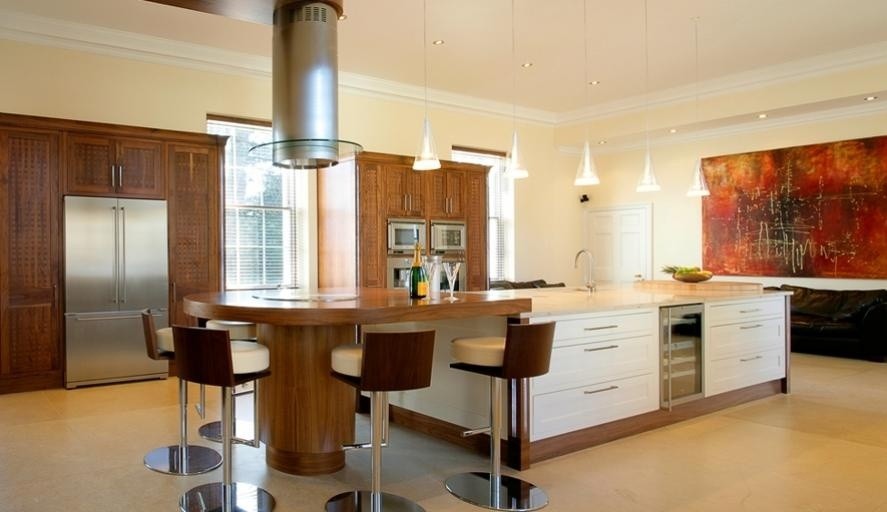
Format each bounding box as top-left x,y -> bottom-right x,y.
420,262 -> 437,301
442,262 -> 461,300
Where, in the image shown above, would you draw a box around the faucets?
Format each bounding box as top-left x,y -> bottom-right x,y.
574,249 -> 598,293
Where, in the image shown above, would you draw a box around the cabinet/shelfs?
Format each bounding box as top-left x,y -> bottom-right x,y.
2,114 -> 229,395
316,152 -> 491,293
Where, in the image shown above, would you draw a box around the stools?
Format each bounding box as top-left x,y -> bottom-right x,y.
198,319 -> 258,444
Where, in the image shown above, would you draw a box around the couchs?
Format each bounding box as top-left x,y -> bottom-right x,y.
765,285 -> 886,363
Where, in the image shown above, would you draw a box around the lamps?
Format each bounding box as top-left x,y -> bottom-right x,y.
572,0 -> 601,187
411,1 -> 445,172
686,2 -> 711,197
635,1 -> 660,192
503,0 -> 530,178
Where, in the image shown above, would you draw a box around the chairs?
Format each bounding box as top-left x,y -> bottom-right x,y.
324,330 -> 436,512
141,307 -> 224,477
444,321 -> 556,512
172,324 -> 275,512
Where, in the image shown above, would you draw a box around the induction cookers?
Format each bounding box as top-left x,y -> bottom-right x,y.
251,288 -> 360,302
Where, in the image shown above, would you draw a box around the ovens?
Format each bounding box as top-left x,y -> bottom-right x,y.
430,220 -> 466,255
388,218 -> 426,255
386,257 -> 427,290
431,257 -> 467,292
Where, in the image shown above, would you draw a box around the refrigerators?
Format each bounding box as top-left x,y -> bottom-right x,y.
63,195 -> 169,389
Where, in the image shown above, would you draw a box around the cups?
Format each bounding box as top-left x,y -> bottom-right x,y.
421,255 -> 442,300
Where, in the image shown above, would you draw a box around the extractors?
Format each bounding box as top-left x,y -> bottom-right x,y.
246,3 -> 364,170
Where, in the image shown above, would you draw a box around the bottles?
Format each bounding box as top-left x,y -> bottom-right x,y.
409,243 -> 427,299
